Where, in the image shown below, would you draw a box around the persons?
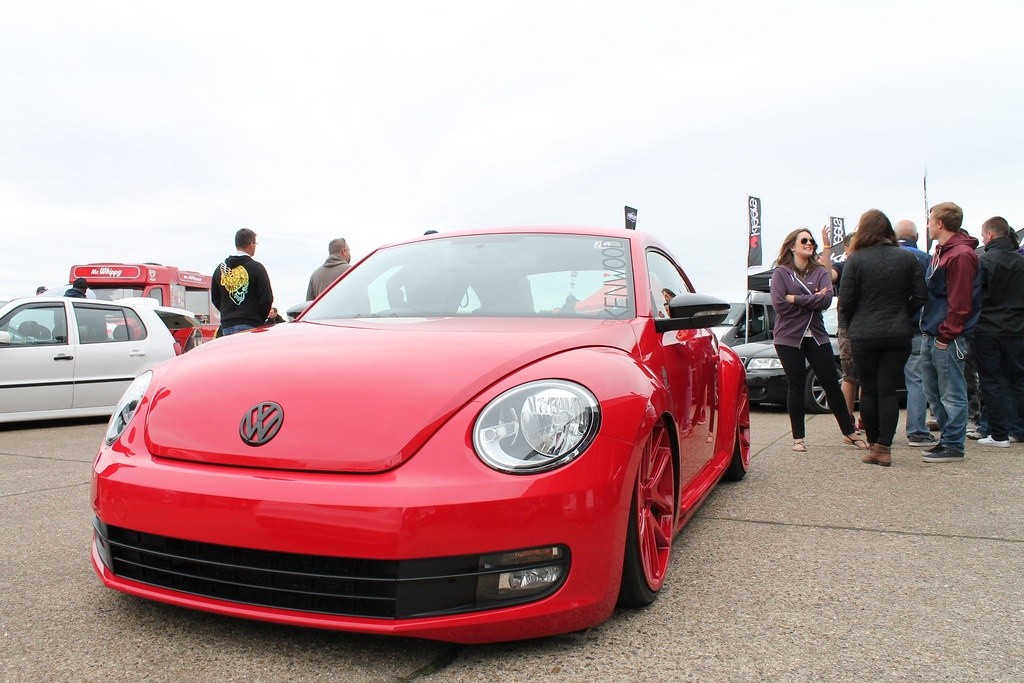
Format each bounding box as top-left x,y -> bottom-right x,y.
658,288 -> 676,318
770,227 -> 870,451
822,224 -> 866,435
561,295 -> 578,313
894,220 -> 940,446
387,230 -> 440,306
54,277 -> 88,329
838,209 -> 928,466
267,307 -> 286,324
919,201 -> 984,463
306,237 -> 371,312
211,228 -> 274,335
36,286 -> 48,295
925,217 -> 1024,448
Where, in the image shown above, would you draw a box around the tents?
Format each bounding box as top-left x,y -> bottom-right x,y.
745,238 -> 847,343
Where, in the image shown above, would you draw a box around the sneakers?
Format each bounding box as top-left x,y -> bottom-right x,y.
1007,435 -> 1016,442
909,433 -> 939,446
921,443 -> 964,462
966,430 -> 983,439
977,434 -> 1011,448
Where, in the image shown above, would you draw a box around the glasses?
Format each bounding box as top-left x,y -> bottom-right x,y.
797,239 -> 815,245
250,242 -> 258,245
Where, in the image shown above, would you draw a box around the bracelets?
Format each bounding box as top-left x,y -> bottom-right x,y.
824,245 -> 831,248
936,340 -> 947,346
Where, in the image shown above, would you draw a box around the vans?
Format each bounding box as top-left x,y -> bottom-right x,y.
709,287 -> 779,347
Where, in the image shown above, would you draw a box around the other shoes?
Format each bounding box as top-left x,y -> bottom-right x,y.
966,420 -> 979,432
857,418 -> 865,432
926,419 -> 939,430
850,415 -> 861,434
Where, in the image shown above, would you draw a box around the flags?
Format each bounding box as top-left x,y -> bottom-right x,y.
748,196 -> 762,268
831,217 -> 845,246
625,206 -> 638,230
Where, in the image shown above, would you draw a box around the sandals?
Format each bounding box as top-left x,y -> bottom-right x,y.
844,435 -> 870,449
793,440 -> 807,451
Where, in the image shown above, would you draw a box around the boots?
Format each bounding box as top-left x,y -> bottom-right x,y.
863,443 -> 892,466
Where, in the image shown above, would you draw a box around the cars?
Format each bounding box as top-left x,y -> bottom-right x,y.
0,295 -> 184,424
91,225 -> 751,646
98,306 -> 203,353
729,295 -> 909,415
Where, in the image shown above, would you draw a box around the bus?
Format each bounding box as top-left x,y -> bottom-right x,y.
69,261 -> 222,342
